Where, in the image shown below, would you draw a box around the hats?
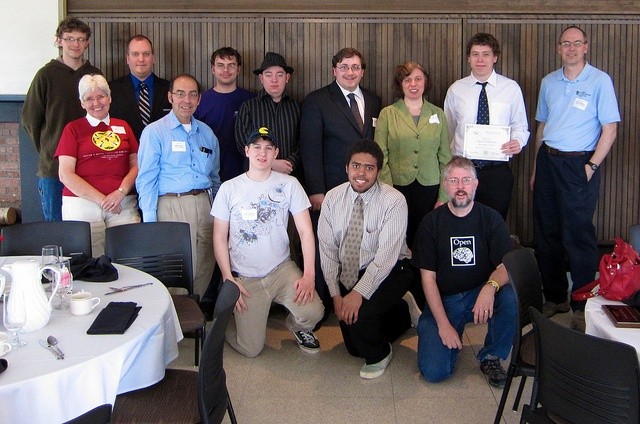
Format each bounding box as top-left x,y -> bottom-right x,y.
246,127 -> 278,147
254,52 -> 294,75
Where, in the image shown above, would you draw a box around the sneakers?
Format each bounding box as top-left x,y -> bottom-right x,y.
570,310 -> 585,332
542,301 -> 570,318
400,290 -> 423,327
480,355 -> 508,388
359,342 -> 393,379
293,327 -> 320,354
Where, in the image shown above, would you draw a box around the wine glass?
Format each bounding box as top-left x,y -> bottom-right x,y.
42,245 -> 59,292
52,259 -> 71,310
3,290 -> 26,349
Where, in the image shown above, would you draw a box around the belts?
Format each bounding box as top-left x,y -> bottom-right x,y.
162,189 -> 208,196
542,142 -> 594,157
473,161 -> 507,167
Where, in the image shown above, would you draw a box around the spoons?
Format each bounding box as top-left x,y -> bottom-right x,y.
47,336 -> 64,356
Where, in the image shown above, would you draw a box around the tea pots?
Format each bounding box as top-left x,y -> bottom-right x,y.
1,260 -> 61,332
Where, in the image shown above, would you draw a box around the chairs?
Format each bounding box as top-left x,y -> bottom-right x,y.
524,306 -> 640,424
1,221 -> 92,260
111,278 -> 240,423
492,247 -> 582,424
105,221 -> 207,369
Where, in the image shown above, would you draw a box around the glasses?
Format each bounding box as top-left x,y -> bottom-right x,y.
60,35 -> 88,42
559,41 -> 587,47
81,94 -> 107,103
171,90 -> 200,98
445,176 -> 475,185
212,63 -> 239,69
335,65 -> 364,70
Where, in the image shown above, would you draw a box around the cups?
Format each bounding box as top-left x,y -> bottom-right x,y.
70,293 -> 100,316
0,332 -> 12,357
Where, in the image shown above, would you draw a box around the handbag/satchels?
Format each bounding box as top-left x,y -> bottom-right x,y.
572,235 -> 640,301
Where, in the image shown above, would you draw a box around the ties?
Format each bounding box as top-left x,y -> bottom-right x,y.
348,93 -> 364,131
477,81 -> 490,170
338,196 -> 364,290
139,81 -> 151,126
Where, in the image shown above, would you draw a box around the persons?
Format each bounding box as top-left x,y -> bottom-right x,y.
107,33 -> 172,145
234,51 -> 300,175
374,61 -> 452,258
191,47 -> 255,183
54,72 -> 141,258
316,140 -> 424,378
443,32 -> 532,222
209,126 -> 325,358
300,48 -> 382,212
530,26 -> 621,333
20,17 -> 104,221
410,157 -> 520,386
135,73 -> 221,304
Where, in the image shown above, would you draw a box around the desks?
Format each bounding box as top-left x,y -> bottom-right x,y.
583,282 -> 640,364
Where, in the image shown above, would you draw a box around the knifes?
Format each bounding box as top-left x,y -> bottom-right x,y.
39,339 -> 64,360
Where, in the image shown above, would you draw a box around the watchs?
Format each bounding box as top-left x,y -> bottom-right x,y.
118,188 -> 127,196
486,281 -> 501,290
586,159 -> 600,172
